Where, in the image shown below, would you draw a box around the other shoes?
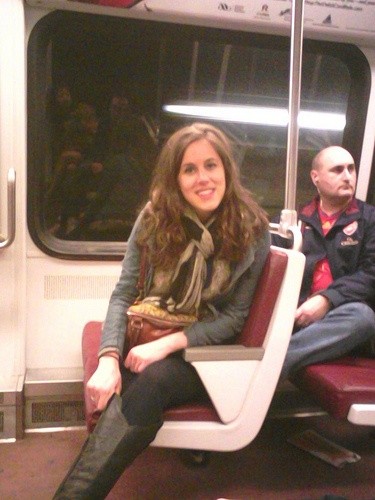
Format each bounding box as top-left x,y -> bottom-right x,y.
191,450 -> 209,471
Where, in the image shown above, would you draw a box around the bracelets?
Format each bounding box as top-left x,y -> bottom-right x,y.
102,354 -> 119,362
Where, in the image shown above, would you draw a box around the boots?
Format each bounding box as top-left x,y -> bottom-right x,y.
52,393 -> 164,500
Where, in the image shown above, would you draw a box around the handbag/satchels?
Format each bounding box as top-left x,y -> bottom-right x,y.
127,245 -> 199,347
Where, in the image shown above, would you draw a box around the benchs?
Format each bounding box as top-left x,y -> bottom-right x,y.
82,224 -> 307,468
288,355 -> 375,427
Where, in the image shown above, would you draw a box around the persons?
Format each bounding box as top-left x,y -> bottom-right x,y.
51,122 -> 272,500
46,80 -> 157,239
176,144 -> 375,472
